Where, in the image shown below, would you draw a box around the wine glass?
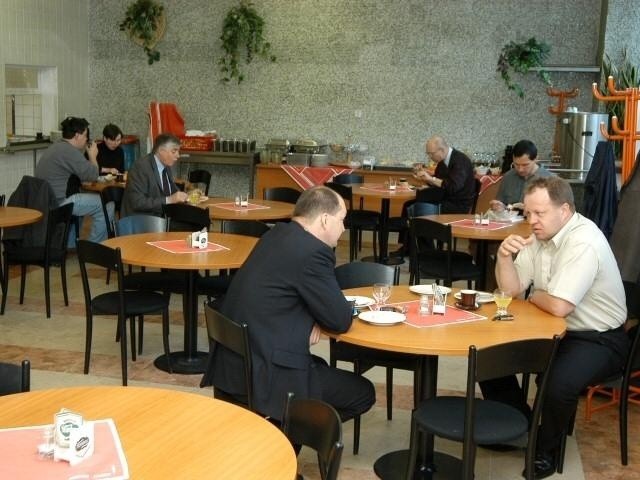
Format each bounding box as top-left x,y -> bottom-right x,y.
373,281 -> 393,303
492,288 -> 513,316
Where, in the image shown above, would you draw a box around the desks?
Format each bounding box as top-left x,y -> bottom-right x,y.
329,279 -> 566,479
425,208 -> 540,276
119,226 -> 263,370
350,177 -> 424,246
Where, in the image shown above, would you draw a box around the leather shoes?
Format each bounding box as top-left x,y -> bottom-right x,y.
390,246 -> 408,257
521,451 -> 558,480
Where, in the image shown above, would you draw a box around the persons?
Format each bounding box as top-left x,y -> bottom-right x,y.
85,123 -> 125,175
478,177 -> 632,479
488,141 -> 554,214
35,116 -> 115,246
200,187 -> 376,479
120,134 -> 188,218
389,136 -> 477,257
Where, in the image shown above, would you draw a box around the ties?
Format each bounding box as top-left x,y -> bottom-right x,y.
162,168 -> 170,197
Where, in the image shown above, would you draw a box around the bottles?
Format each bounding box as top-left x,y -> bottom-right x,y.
384,175 -> 409,190
431,288 -> 447,315
419,295 -> 429,314
234,193 -> 248,207
472,151 -> 499,167
472,213 -> 490,226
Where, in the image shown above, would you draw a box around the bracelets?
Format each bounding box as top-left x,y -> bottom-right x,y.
431,177 -> 436,183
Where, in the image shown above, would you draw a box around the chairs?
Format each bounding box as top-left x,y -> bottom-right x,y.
186,194 -> 300,226
263,183 -> 305,210
70,236 -> 178,386
199,302 -> 274,428
93,185 -> 129,231
114,212 -> 171,342
405,220 -> 471,285
272,393 -> 356,480
1,202 -> 74,319
182,170 -> 220,198
161,201 -> 212,235
332,258 -> 408,383
405,339 -> 555,479
559,269 -> 635,472
324,182 -> 378,256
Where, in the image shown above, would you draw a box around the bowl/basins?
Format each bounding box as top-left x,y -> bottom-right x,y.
475,166 -> 502,176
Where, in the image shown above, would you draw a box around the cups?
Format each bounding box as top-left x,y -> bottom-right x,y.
189,191 -> 201,205
36,131 -> 42,138
116,173 -> 124,183
211,138 -> 256,152
461,289 -> 479,306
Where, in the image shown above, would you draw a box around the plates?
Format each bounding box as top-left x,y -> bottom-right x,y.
454,289 -> 494,303
186,195 -> 209,202
358,310 -> 406,326
408,184 -> 429,190
455,302 -> 480,310
344,295 -> 374,309
409,284 -> 454,295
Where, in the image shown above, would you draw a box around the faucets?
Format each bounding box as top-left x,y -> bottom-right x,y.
552,150 -> 561,161
